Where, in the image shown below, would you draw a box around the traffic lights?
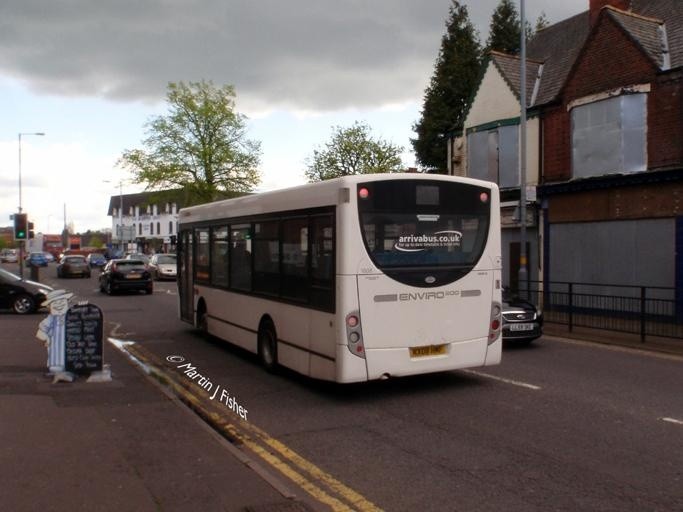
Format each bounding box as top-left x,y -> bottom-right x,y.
28,222 -> 35,237
15,214 -> 26,239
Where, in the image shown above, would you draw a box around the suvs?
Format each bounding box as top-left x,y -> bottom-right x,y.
56,255 -> 90,278
98,259 -> 152,295
0,249 -> 16,263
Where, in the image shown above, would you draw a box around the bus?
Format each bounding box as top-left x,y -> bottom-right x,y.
174,172 -> 501,386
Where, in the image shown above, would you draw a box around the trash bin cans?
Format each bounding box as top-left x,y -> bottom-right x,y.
30,265 -> 39,282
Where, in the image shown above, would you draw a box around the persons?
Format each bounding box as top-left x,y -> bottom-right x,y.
222,239 -> 256,289
34,289 -> 88,377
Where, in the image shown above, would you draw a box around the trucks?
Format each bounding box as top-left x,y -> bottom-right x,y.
43,234 -> 64,260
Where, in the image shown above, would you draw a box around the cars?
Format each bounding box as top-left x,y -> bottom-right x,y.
0,269 -> 53,314
24,251 -> 48,267
501,281 -> 545,344
46,253 -> 53,262
125,254 -> 149,265
149,254 -> 177,280
86,254 -> 106,267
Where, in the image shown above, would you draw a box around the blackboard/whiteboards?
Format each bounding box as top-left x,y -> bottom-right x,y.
65,302 -> 105,373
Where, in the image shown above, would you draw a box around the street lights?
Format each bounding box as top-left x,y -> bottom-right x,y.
103,178 -> 123,250
16,131 -> 47,214
